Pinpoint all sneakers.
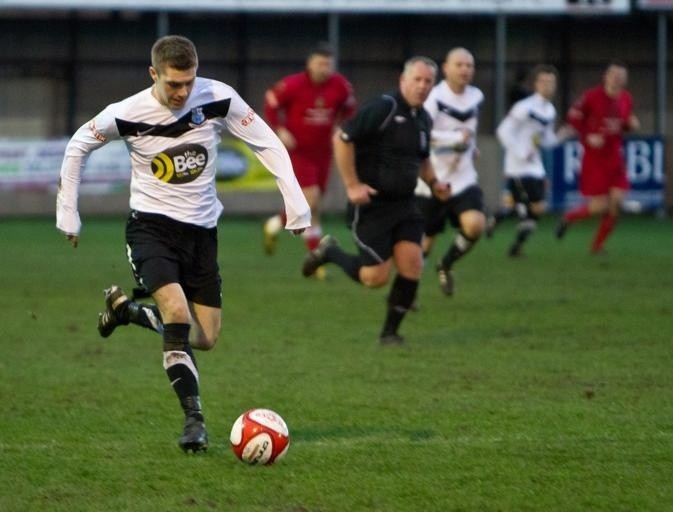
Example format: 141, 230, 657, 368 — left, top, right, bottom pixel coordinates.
263, 221, 417, 345
97, 285, 129, 338
435, 258, 454, 296
178, 412, 208, 454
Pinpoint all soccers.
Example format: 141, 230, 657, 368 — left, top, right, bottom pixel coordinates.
231, 408, 289, 466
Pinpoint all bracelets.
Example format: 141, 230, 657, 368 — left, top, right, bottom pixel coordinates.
427, 178, 437, 189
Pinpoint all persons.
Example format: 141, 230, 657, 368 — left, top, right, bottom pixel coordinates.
302, 56, 452, 349
551, 64, 643, 256
56, 36, 313, 453
409, 45, 484, 312
486, 65, 576, 260
261, 50, 359, 282
